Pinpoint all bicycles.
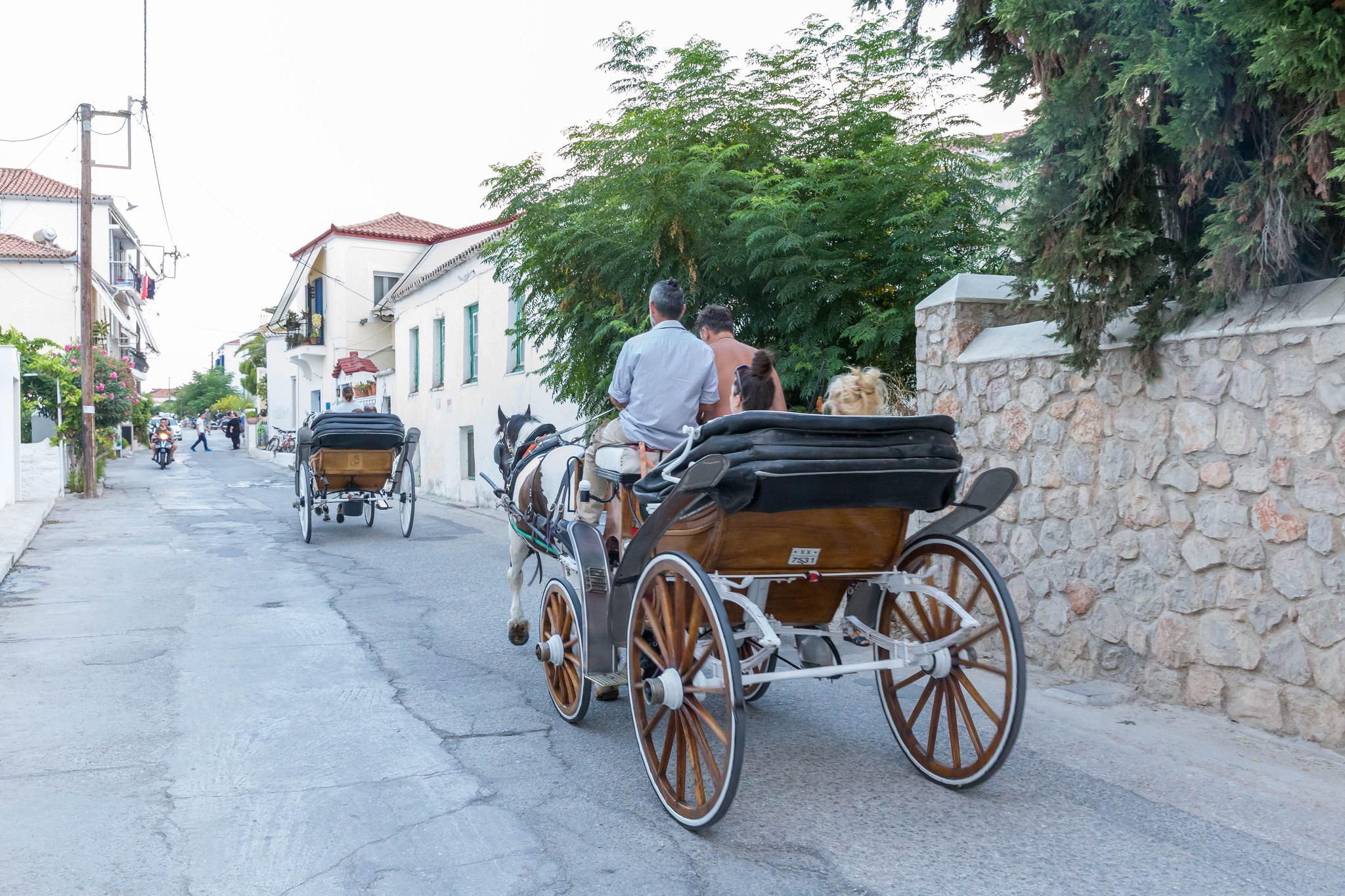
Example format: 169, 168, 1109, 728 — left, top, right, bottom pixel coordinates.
265, 426, 298, 454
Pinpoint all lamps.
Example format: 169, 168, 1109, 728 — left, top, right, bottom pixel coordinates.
336, 385, 340, 397
360, 318, 368, 325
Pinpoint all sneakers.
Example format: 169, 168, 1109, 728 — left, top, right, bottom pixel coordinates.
190, 447, 197, 452
204, 448, 212, 452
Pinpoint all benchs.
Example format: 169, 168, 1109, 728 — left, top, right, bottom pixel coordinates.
309, 448, 394, 491
639, 404, 919, 572
598, 445, 669, 495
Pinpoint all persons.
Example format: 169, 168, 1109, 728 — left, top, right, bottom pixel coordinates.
240, 411, 246, 446
227, 411, 241, 450
156, 412, 163, 417
793, 574, 871, 648
557, 277, 720, 567
821, 369, 887, 416
695, 304, 787, 427
183, 415, 190, 426
729, 350, 776, 415
150, 418, 177, 461
352, 405, 378, 413
204, 408, 212, 436
334, 386, 365, 413
190, 412, 212, 451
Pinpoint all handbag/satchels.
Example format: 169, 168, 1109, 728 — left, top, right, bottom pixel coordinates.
238, 418, 243, 433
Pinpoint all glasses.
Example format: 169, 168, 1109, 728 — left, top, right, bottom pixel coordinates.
734, 365, 752, 402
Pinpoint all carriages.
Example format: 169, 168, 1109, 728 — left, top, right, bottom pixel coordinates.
476, 402, 1027, 831
292, 408, 420, 544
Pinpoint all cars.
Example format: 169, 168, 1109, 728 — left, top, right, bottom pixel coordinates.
159, 412, 178, 423
150, 417, 182, 441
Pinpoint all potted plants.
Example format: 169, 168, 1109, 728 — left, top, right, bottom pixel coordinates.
363, 382, 373, 396
352, 382, 360, 396
284, 333, 310, 348
307, 324, 320, 344
358, 381, 368, 398
366, 377, 376, 395
300, 307, 310, 318
246, 411, 258, 424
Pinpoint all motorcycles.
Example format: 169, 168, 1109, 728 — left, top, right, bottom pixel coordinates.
191, 407, 243, 433
149, 428, 180, 470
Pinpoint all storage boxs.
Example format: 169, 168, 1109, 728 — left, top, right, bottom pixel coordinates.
116, 281, 132, 286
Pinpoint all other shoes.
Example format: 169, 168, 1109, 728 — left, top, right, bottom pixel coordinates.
209, 434, 211, 435
231, 448, 236, 450
151, 457, 155, 459
171, 459, 174, 461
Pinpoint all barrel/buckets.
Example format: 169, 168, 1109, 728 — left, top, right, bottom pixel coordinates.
341, 484, 364, 517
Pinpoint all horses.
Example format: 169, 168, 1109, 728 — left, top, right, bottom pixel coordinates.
494, 404, 620, 701
303, 409, 344, 523
